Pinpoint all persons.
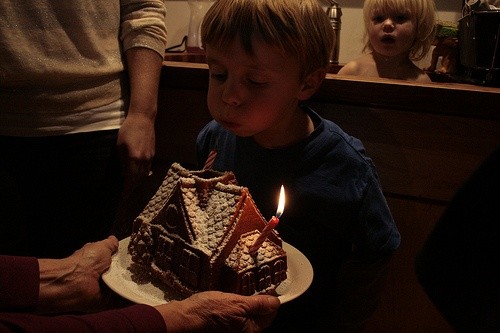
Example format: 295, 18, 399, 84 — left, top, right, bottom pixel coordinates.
0, 235, 279, 333
0, 0, 168, 261
195, 0, 401, 333
337, 0, 437, 83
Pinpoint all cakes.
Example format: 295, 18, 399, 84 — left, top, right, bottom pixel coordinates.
126, 163, 290, 303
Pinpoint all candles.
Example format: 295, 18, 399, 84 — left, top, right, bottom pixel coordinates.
247, 185, 285, 254
205, 148, 219, 170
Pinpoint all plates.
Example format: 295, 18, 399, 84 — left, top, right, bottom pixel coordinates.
101, 235, 314, 307
